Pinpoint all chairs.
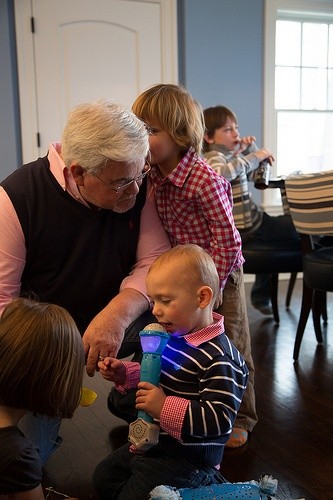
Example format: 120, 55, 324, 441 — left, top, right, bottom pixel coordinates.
228, 165, 333, 361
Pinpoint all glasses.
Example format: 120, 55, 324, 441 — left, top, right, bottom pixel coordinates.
94, 158, 151, 193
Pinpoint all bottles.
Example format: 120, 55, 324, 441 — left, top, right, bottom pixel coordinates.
252, 159, 272, 190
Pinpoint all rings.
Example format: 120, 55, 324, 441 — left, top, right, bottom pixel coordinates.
99, 356, 105, 361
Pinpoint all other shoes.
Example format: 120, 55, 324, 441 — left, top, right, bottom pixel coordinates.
107, 387, 138, 423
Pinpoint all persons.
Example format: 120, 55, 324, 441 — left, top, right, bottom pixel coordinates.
0, 299, 85, 500
92, 243, 250, 499
201, 105, 333, 315
0, 100, 171, 471
130, 83, 259, 447
